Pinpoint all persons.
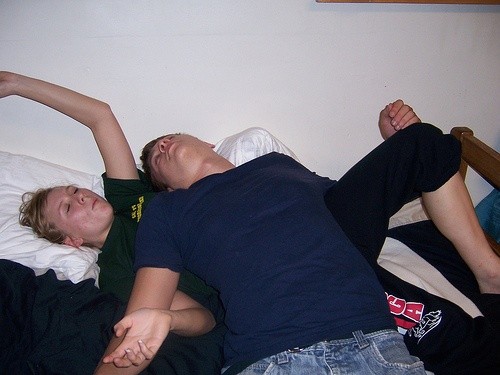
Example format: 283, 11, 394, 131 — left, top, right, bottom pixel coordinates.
102, 99, 431, 375
1, 70, 500, 375
